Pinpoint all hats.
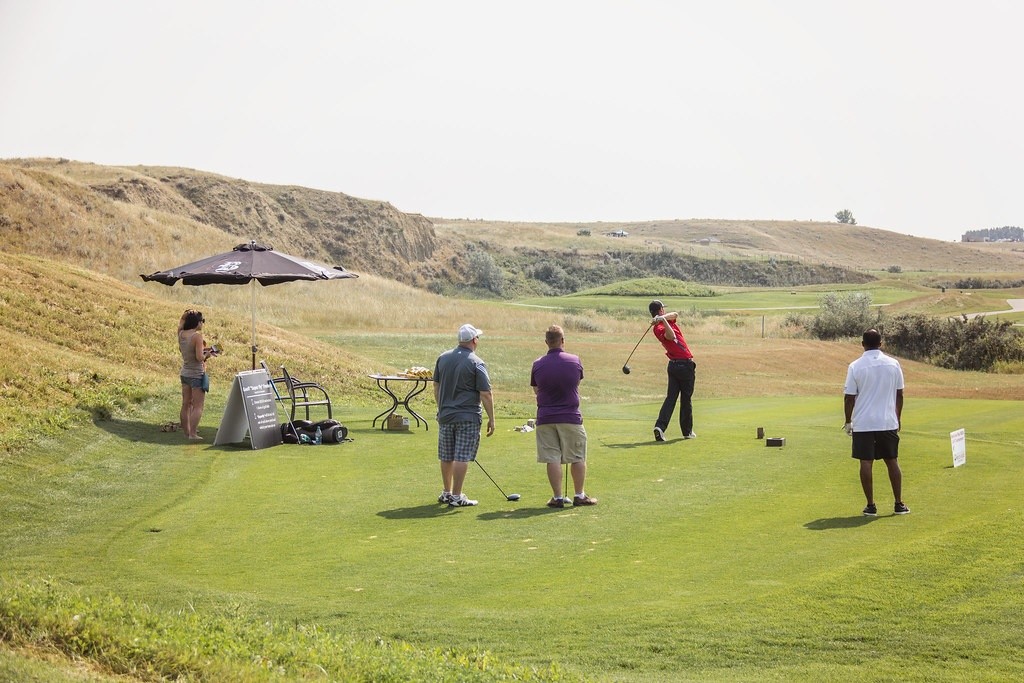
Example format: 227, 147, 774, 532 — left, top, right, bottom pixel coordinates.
649, 300, 667, 311
458, 324, 482, 342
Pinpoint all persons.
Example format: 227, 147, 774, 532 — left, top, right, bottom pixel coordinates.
844, 329, 910, 516
530, 325, 597, 507
433, 324, 495, 507
178, 309, 222, 440
649, 300, 697, 442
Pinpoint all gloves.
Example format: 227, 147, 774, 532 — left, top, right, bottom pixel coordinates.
844, 422, 852, 436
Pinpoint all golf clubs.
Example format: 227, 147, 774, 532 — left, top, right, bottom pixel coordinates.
436, 412, 522, 500
622, 314, 658, 374
259, 360, 301, 445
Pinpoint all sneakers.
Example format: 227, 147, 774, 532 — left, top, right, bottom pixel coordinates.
438, 491, 451, 503
684, 431, 696, 438
448, 495, 479, 506
894, 502, 910, 514
573, 496, 598, 506
863, 504, 877, 515
654, 427, 665, 441
546, 497, 564, 508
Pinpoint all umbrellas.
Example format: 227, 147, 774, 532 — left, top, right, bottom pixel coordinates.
139, 241, 360, 370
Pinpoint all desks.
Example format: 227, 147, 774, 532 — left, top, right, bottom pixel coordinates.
367, 375, 434, 431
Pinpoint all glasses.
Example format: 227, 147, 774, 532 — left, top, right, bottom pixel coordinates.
199, 318, 205, 323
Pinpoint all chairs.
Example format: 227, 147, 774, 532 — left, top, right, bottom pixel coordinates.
260, 361, 310, 421
279, 365, 332, 422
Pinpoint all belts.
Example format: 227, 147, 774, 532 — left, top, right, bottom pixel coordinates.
670, 358, 694, 363
